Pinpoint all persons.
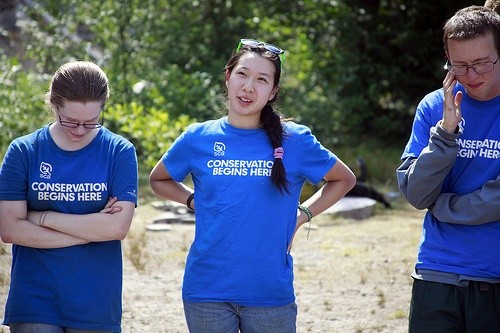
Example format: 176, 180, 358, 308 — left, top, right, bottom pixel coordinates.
396, 5, 500, 333
148, 35, 357, 333
0, 60, 138, 333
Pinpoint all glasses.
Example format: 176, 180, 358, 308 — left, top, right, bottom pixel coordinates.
59, 110, 104, 128
445, 55, 500, 75
237, 39, 284, 62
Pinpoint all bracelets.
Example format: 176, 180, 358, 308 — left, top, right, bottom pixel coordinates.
297, 200, 313, 222
38, 209, 50, 228
186, 190, 195, 210
